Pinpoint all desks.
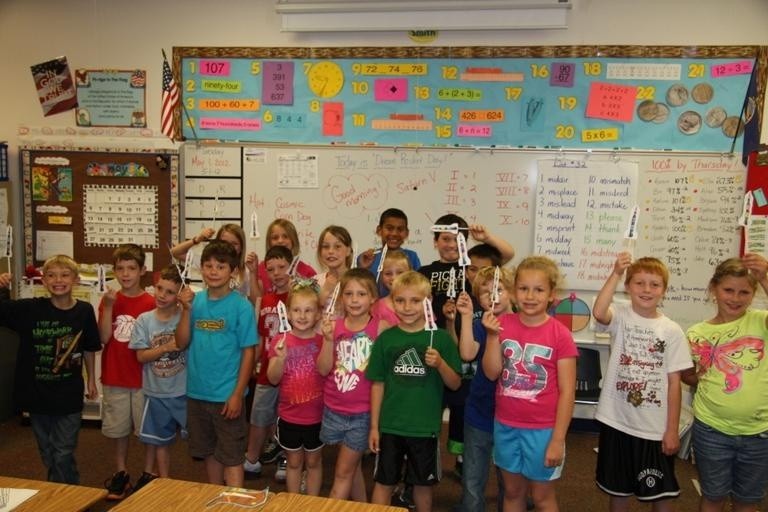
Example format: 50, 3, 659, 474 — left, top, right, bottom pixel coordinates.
0, 476, 109, 512
109, 475, 409, 512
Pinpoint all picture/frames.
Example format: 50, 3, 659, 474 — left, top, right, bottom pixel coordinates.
74, 69, 147, 127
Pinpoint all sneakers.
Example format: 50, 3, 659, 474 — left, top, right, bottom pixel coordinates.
132, 472, 159, 496
192, 448, 204, 459
107, 470, 130, 498
454, 458, 463, 479
244, 438, 308, 495
399, 482, 416, 508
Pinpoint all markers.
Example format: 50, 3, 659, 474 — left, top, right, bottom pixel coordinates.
360, 141, 378, 147
490, 143, 511, 149
331, 141, 351, 146
654, 148, 673, 151
613, 145, 632, 150
433, 143, 451, 147
453, 142, 477, 148
518, 144, 537, 149
405, 142, 423, 147
544, 144, 564, 150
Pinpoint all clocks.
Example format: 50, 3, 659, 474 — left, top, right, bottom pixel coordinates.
308, 61, 344, 97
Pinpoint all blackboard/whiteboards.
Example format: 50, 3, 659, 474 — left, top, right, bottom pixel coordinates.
178, 140, 768, 343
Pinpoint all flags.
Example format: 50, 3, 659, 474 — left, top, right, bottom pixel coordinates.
160, 58, 182, 144
742, 60, 760, 166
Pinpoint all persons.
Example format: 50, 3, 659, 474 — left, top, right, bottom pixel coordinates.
682, 253, 768, 512
592, 251, 694, 512
1, 255, 102, 485
96, 209, 579, 511
